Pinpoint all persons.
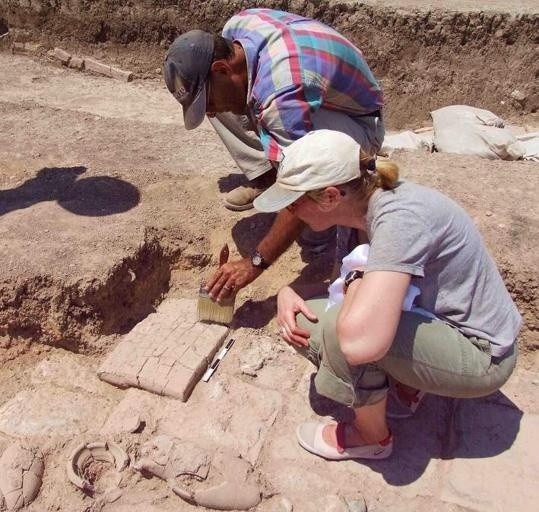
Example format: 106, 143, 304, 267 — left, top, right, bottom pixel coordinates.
271, 129, 524, 461
164, 7, 385, 304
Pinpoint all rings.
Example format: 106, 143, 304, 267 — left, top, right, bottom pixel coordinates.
279, 327, 286, 334
223, 283, 231, 292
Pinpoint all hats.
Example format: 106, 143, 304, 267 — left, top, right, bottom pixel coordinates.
253, 128, 368, 214
162, 29, 214, 129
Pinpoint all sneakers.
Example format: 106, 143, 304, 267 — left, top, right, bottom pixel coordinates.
300, 224, 339, 245
296, 418, 394, 461
224, 172, 276, 212
387, 379, 426, 420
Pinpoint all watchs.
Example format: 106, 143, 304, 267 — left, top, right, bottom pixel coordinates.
248, 248, 269, 271
343, 269, 365, 287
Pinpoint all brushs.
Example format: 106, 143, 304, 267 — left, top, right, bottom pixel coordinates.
197, 242, 237, 323
